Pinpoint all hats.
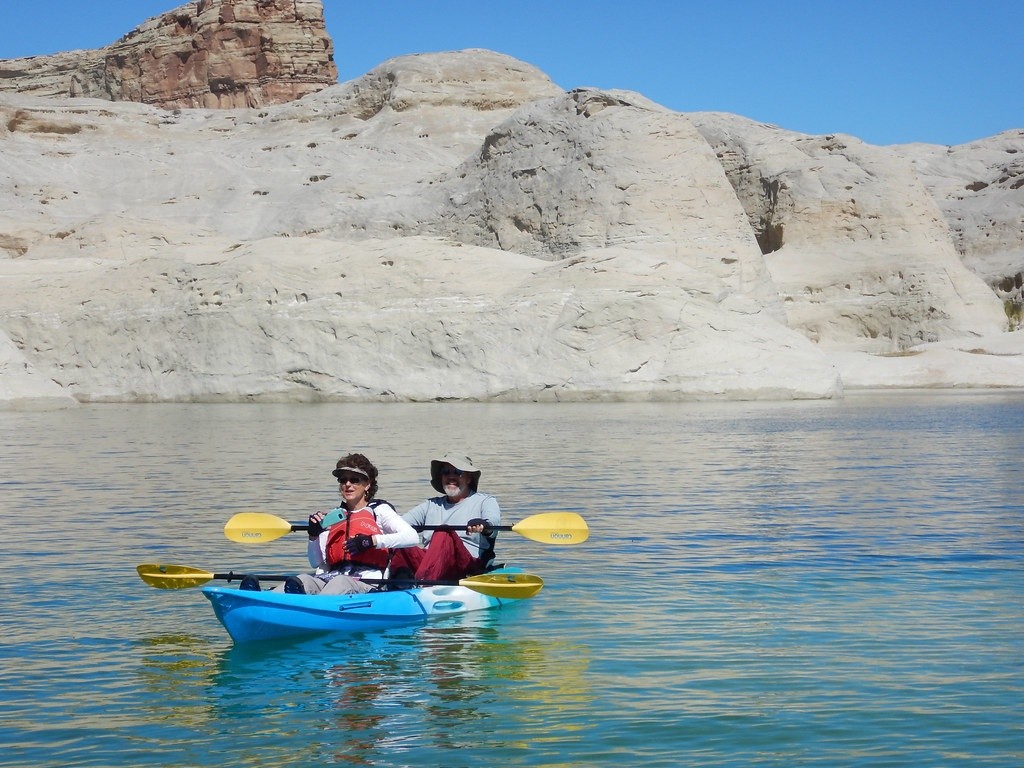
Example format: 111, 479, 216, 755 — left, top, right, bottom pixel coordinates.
430, 450, 481, 494
332, 463, 369, 483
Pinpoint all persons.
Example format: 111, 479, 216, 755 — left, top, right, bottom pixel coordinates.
389, 453, 501, 588
239, 453, 419, 594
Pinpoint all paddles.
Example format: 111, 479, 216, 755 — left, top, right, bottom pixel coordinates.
224, 510, 590, 546
136, 562, 544, 600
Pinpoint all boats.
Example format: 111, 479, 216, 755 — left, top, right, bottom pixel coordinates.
201, 568, 530, 644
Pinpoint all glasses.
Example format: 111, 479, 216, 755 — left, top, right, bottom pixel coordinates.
337, 477, 369, 484
439, 466, 470, 477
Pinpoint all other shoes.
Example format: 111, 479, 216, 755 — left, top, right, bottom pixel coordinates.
239, 575, 261, 591
389, 568, 413, 591
284, 576, 305, 594
